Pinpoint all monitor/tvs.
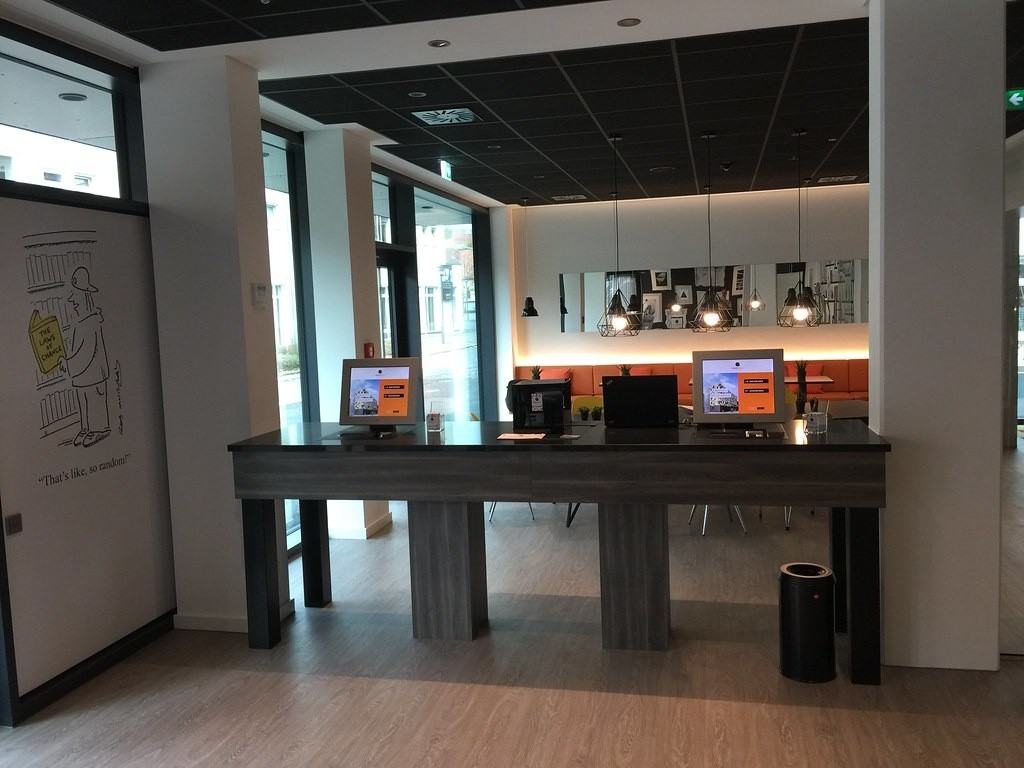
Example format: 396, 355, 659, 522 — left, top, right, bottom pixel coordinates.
692, 349, 788, 440
340, 357, 418, 440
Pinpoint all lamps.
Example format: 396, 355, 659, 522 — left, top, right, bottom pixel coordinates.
785, 281, 799, 307
744, 265, 766, 312
686, 191, 735, 333
626, 272, 641, 314
596, 196, 642, 338
519, 198, 539, 317
777, 186, 823, 328
809, 282, 831, 325
663, 270, 686, 316
798, 188, 819, 308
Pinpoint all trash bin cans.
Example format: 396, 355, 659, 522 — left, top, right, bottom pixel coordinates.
776, 563, 838, 684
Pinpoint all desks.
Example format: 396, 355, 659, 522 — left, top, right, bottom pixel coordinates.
688, 375, 834, 387
787, 400, 869, 421
226, 418, 891, 685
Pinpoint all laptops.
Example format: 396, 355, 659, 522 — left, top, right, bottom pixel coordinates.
602, 374, 679, 429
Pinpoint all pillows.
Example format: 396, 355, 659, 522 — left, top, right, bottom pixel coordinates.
538, 368, 571, 379
786, 366, 826, 394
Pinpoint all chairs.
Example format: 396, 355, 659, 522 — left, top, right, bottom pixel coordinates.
571, 397, 605, 416
679, 405, 733, 536
783, 386, 797, 405
470, 412, 535, 520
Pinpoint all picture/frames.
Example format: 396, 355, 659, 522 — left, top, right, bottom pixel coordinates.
732, 266, 744, 296
695, 267, 725, 287
651, 269, 672, 290
675, 286, 693, 305
696, 290, 730, 312
642, 293, 663, 323
737, 298, 744, 317
665, 309, 687, 329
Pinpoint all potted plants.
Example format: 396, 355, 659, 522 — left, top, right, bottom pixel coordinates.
617, 364, 634, 376
591, 406, 604, 420
794, 393, 806, 412
577, 407, 590, 420
529, 365, 543, 379
810, 396, 819, 411
794, 359, 808, 378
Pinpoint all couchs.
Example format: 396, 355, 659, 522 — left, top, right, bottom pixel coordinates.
593, 363, 674, 399
515, 365, 593, 402
675, 364, 692, 406
784, 360, 854, 413
849, 359, 870, 412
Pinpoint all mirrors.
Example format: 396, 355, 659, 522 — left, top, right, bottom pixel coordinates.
559, 260, 870, 333
